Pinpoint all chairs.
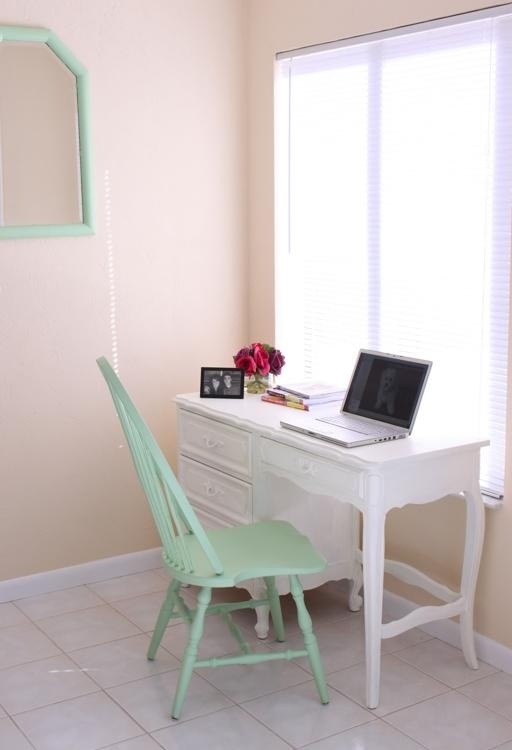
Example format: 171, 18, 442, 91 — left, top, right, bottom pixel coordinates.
96, 357, 332, 720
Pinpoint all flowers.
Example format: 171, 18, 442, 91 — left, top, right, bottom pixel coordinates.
233, 343, 284, 393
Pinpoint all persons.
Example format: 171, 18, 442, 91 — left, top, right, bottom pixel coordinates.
204, 372, 240, 396
206, 373, 225, 396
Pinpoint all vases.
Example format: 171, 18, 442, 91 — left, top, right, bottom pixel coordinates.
244, 376, 273, 391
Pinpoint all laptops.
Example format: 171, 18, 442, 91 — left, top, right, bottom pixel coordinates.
279, 347, 433, 449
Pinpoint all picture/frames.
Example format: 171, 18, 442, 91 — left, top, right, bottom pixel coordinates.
199, 367, 245, 398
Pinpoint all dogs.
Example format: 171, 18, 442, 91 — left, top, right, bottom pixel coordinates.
373, 367, 399, 416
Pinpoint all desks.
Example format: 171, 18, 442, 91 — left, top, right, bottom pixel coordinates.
172, 379, 496, 711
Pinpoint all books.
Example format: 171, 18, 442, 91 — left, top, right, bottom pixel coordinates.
261, 393, 344, 412
266, 387, 344, 404
277, 381, 348, 399
